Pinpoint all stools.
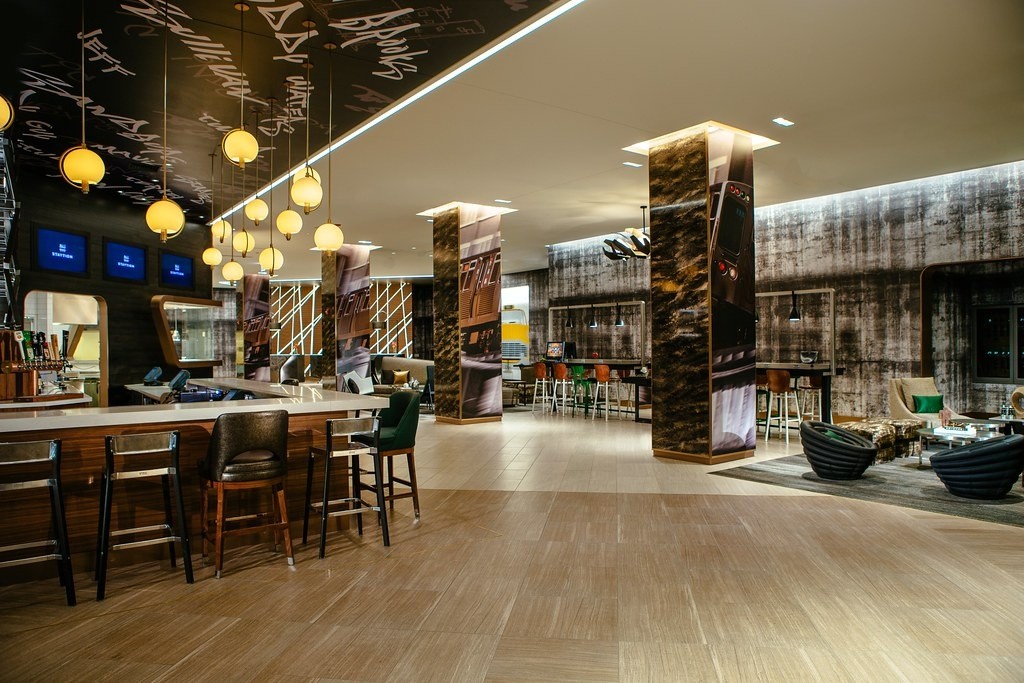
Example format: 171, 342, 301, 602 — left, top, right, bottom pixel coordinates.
504, 358, 833, 444
0, 380, 419, 606
833, 418, 928, 465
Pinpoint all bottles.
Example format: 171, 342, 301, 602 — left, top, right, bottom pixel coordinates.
1001, 405, 1007, 419
410, 377, 414, 386
1009, 405, 1013, 419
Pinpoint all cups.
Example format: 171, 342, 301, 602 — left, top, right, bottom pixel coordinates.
403, 383, 408, 388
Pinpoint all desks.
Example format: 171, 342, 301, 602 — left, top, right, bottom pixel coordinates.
124, 382, 171, 404
915, 428, 1005, 466
0, 378, 390, 588
546, 359, 648, 422
756, 363, 831, 424
988, 416, 1024, 435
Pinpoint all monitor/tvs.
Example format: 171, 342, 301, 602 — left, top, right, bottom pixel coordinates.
546, 340, 565, 361
158, 248, 196, 292
30, 222, 91, 278
102, 236, 149, 285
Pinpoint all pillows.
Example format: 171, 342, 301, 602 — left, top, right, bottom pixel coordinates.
901, 377, 939, 412
381, 369, 410, 385
912, 395, 943, 413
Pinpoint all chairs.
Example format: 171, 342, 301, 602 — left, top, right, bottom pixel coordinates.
421, 365, 435, 413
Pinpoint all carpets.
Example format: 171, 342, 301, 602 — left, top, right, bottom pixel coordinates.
708, 439, 1024, 528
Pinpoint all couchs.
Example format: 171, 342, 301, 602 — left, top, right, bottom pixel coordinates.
800, 420, 878, 480
890, 377, 1024, 499
372, 356, 435, 394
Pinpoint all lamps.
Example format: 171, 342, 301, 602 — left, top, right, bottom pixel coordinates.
172, 309, 181, 341
564, 205, 801, 328
0, 0, 344, 287
